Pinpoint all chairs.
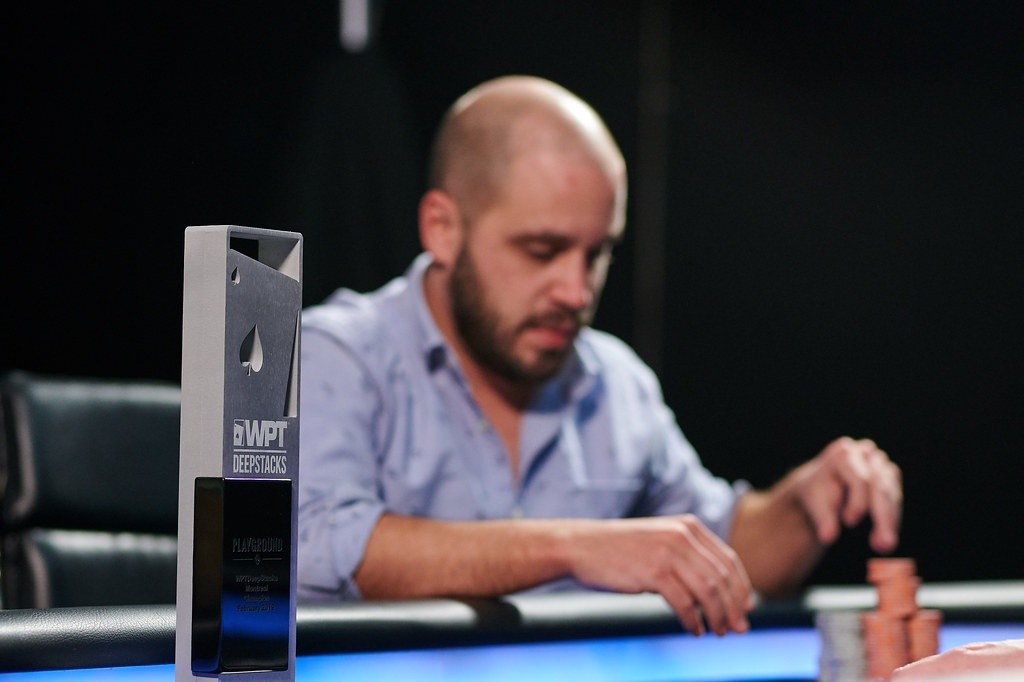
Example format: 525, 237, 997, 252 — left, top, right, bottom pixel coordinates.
0, 373, 205, 608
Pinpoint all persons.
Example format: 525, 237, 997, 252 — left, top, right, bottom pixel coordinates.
294, 72, 902, 639
